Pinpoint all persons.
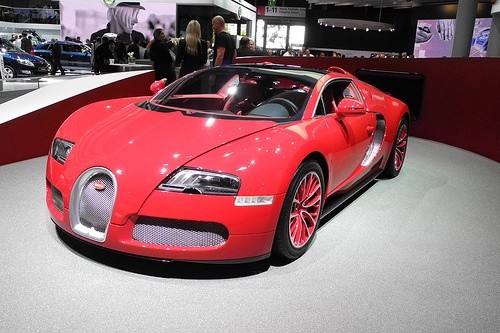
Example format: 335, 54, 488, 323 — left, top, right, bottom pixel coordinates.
175, 20, 208, 78
90, 0, 145, 41
144, 29, 176, 86
10, 25, 414, 74
416, 19, 453, 44
209, 16, 237, 94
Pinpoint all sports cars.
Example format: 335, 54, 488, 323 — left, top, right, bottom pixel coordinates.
46, 64, 411, 265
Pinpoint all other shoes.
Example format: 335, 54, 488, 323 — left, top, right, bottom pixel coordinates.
50, 73, 55, 75
61, 73, 65, 75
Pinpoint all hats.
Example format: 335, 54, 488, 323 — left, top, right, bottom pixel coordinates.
103, 0, 146, 10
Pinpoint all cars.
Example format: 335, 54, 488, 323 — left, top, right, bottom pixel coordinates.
23, 30, 94, 73
0, 38, 48, 79
